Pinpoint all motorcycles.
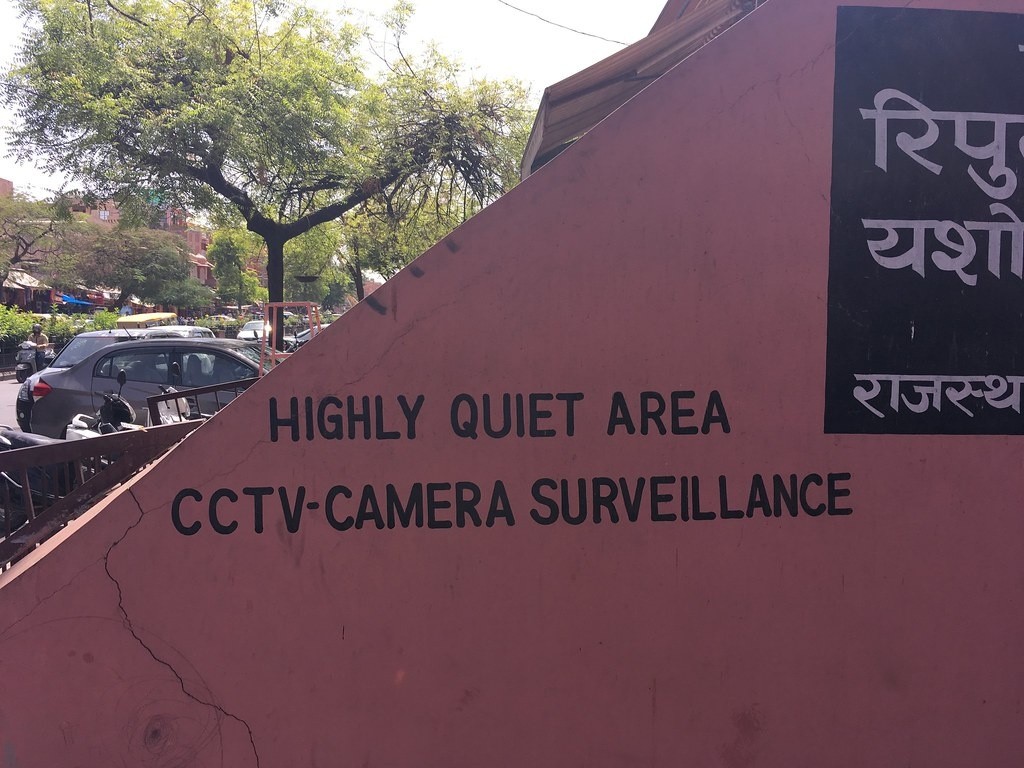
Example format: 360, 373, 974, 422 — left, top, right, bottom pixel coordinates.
116, 312, 178, 331
0, 369, 147, 521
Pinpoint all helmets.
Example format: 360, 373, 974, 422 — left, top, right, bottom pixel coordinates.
32, 323, 43, 333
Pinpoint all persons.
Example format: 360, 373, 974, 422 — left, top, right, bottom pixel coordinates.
28, 324, 49, 372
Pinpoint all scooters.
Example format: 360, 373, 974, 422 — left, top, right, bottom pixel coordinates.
142, 361, 195, 424
16, 340, 58, 383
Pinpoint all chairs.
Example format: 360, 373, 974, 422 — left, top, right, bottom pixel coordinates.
101, 361, 165, 382
185, 354, 212, 386
216, 358, 236, 384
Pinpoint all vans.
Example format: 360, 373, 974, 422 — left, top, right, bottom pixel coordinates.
147, 326, 218, 372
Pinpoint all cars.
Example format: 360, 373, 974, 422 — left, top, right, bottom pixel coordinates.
235, 320, 270, 343
30, 337, 295, 439
296, 324, 331, 342
15, 329, 192, 435
205, 316, 236, 323
